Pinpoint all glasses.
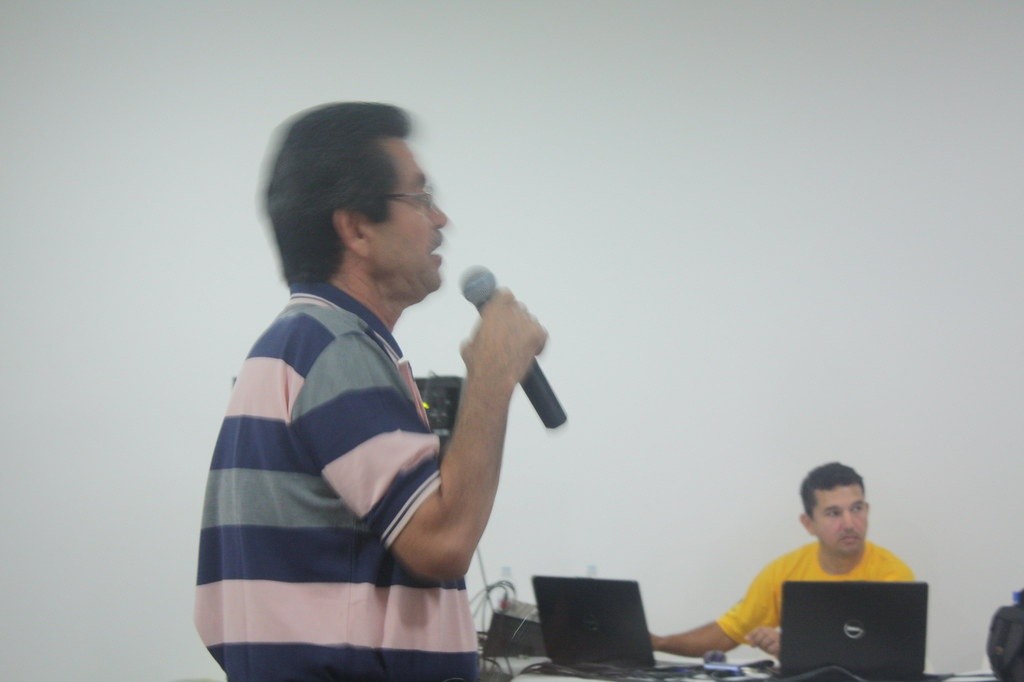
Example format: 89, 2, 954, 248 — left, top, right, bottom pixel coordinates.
339, 185, 435, 211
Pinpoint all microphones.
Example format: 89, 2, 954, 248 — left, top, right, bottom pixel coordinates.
460, 265, 568, 429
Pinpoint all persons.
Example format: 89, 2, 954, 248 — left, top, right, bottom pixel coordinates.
986, 589, 1024, 682
649, 461, 917, 659
193, 100, 546, 682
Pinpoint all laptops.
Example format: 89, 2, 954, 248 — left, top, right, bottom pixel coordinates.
532, 576, 705, 672
759, 580, 930, 682
